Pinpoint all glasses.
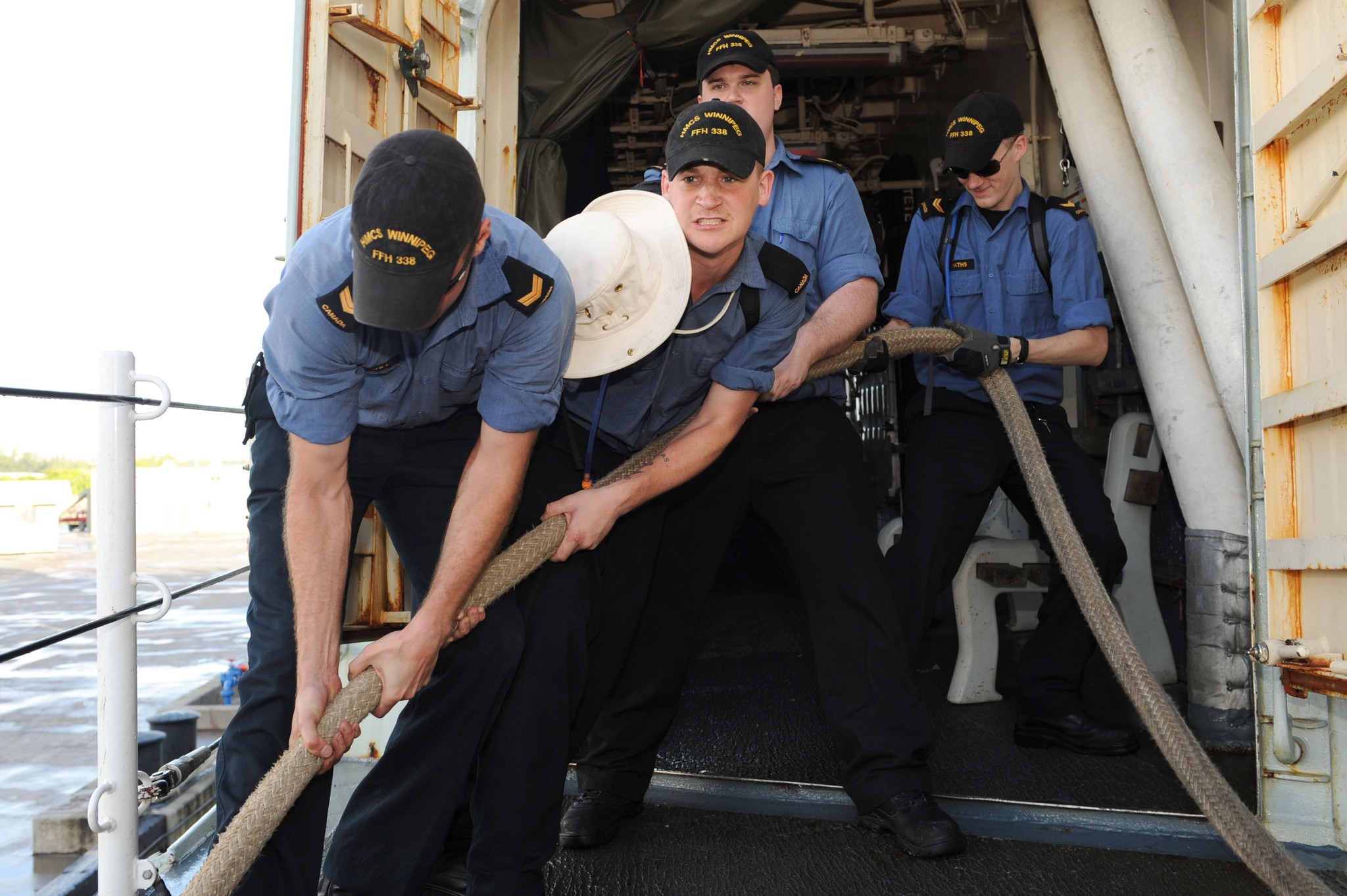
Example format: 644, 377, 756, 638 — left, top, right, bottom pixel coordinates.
949, 133, 1023, 179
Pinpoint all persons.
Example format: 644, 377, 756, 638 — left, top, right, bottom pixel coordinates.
855, 88, 1130, 859
200, 128, 580, 892
447, 100, 812, 894
548, 29, 965, 866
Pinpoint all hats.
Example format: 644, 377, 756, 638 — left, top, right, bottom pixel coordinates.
697, 29, 781, 85
350, 129, 486, 333
665, 98, 767, 182
540, 190, 693, 379
942, 89, 1024, 172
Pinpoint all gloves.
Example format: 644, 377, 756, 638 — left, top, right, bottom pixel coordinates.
847, 331, 890, 377
933, 318, 1013, 381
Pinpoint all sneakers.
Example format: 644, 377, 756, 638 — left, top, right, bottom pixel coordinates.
1013, 708, 1140, 757
558, 789, 644, 851
231, 862, 469, 896
856, 787, 962, 857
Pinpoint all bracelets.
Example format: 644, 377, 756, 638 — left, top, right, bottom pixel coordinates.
1011, 335, 1029, 367
